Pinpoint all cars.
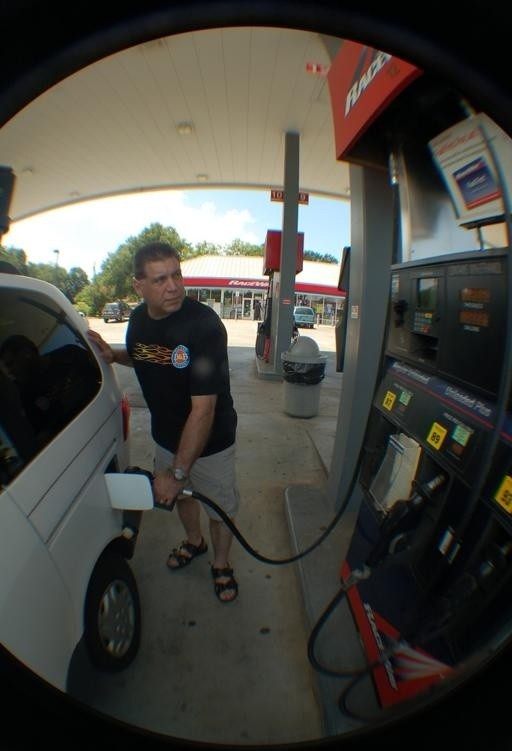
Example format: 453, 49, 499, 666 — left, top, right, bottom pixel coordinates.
1, 272, 155, 706
293, 306, 315, 328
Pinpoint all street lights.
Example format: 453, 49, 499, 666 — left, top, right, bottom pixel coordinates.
53, 249, 59, 265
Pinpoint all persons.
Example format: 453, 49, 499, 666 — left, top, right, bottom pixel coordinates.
87, 242, 241, 604
253, 300, 264, 322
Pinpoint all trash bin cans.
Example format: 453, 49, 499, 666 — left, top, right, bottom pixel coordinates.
281, 336, 328, 417
230, 310, 235, 319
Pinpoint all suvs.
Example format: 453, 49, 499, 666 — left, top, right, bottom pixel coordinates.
102, 301, 132, 323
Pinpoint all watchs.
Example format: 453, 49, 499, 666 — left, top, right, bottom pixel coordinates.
166, 465, 187, 483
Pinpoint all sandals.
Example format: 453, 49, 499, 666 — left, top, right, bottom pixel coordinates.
166, 535, 208, 569
212, 562, 237, 602
